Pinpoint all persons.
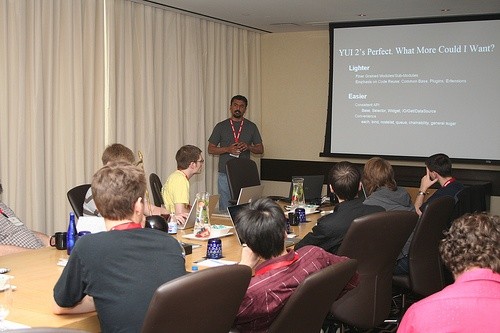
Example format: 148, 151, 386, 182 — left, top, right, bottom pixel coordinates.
294, 160, 386, 253
0, 183, 55, 256
207, 96, 264, 212
414, 153, 461, 215
160, 144, 205, 216
397, 211, 499, 333
362, 157, 419, 275
231, 196, 361, 333
52, 162, 187, 333
82, 143, 188, 226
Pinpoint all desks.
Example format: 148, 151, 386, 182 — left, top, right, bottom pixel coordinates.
0, 200, 341, 333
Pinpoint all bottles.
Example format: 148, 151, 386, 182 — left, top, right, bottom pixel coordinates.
207, 238, 223, 258
295, 208, 306, 223
66, 211, 77, 254
286, 218, 290, 233
167, 214, 178, 234
289, 212, 300, 226
191, 266, 198, 272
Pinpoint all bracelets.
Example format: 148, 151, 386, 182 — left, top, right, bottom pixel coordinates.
418, 191, 426, 196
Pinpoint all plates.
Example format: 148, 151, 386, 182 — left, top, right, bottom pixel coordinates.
284, 210, 319, 216
182, 232, 234, 241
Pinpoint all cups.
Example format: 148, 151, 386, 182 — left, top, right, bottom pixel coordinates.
291, 178, 305, 210
194, 192, 210, 237
330, 193, 338, 203
49, 232, 66, 250
78, 231, 91, 238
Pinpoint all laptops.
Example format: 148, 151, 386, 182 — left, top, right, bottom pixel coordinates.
282, 174, 325, 203
227, 202, 302, 249
177, 198, 197, 229
213, 185, 263, 218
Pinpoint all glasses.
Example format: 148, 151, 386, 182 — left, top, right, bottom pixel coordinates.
189, 159, 204, 164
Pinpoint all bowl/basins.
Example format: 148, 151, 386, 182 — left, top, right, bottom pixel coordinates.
210, 225, 234, 233
305, 205, 320, 210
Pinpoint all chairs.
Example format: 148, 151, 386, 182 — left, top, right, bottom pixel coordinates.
67, 174, 489, 333
